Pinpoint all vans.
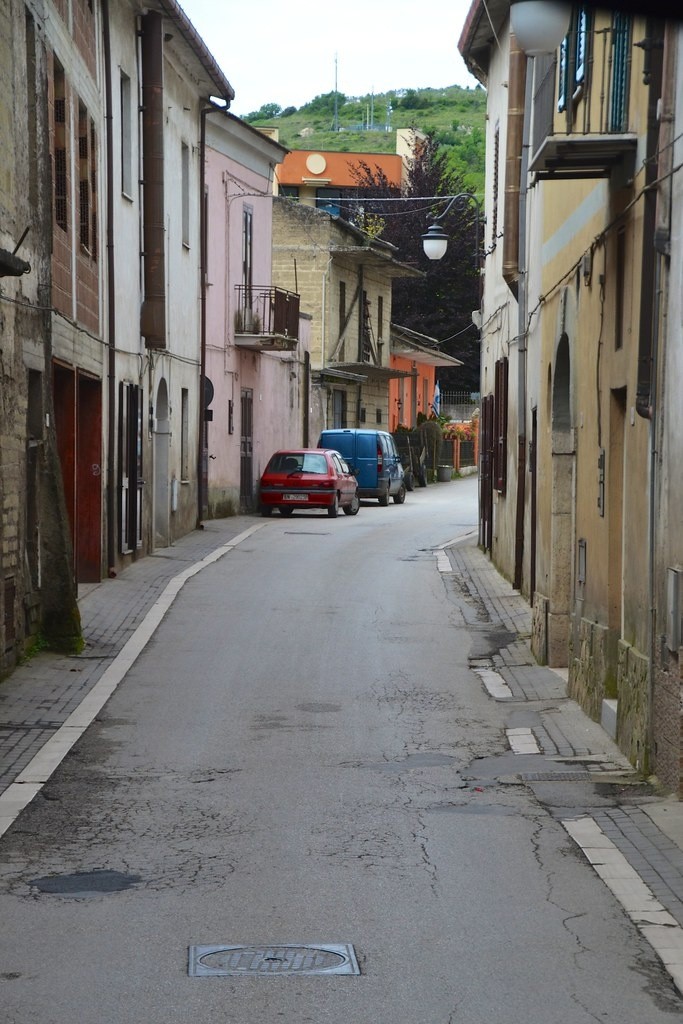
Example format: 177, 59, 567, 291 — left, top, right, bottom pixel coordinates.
317, 428, 406, 505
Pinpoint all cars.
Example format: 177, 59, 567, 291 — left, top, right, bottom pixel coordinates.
260, 447, 360, 517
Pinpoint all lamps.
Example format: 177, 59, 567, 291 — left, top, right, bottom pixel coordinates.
395, 398, 403, 411
508, 0, 571, 56
421, 192, 482, 273
